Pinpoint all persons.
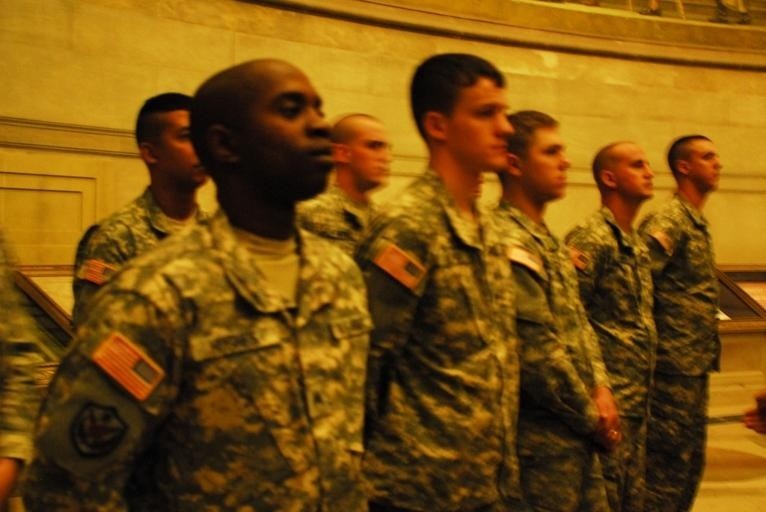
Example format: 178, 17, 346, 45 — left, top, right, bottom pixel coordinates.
1, 52, 724, 512
